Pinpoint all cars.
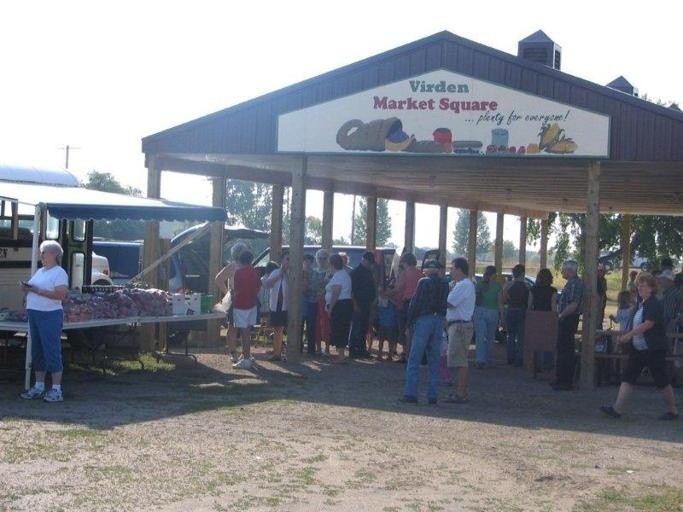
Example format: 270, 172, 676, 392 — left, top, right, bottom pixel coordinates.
441, 271, 561, 349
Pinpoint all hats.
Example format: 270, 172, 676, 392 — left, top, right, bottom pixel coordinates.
423, 258, 440, 269
363, 251, 375, 263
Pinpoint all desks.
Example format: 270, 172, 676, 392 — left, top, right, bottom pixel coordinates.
136, 312, 227, 380
0, 311, 136, 380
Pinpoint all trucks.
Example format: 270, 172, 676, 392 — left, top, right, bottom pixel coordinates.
597, 248, 655, 272
0, 166, 114, 350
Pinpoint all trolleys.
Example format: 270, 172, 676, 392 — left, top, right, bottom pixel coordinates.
570, 315, 616, 386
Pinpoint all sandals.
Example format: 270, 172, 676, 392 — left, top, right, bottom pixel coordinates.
401, 393, 472, 406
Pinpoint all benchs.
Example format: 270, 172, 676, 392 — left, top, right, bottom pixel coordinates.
568, 331, 683, 388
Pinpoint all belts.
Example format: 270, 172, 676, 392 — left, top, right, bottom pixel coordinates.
447, 320, 470, 325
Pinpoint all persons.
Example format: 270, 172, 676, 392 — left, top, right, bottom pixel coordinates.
18, 240, 69, 403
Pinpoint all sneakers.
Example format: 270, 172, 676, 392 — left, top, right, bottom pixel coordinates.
599, 406, 622, 418
657, 411, 679, 421
230, 353, 252, 369
548, 382, 569, 391
43, 389, 64, 402
20, 386, 45, 400
265, 343, 408, 367
473, 359, 554, 376
